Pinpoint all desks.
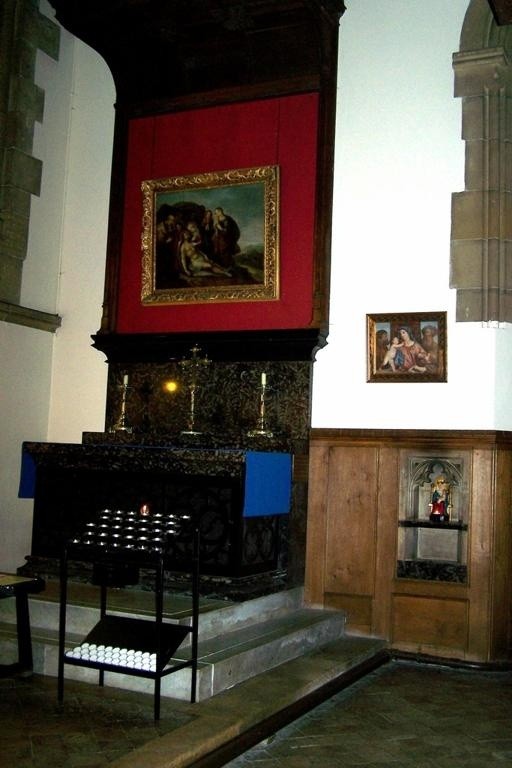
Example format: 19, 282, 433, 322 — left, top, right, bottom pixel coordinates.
0, 571, 47, 676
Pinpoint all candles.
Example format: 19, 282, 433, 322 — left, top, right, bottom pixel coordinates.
261, 373, 267, 385
124, 374, 130, 385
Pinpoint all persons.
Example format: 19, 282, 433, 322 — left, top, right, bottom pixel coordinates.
155, 207, 241, 289
428, 476, 450, 522
375, 326, 438, 372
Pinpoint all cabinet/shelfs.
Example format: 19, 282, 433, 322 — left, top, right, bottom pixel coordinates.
17, 432, 301, 598
394, 446, 470, 586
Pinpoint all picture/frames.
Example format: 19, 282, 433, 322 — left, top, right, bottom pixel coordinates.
365, 309, 448, 385
140, 164, 281, 305
